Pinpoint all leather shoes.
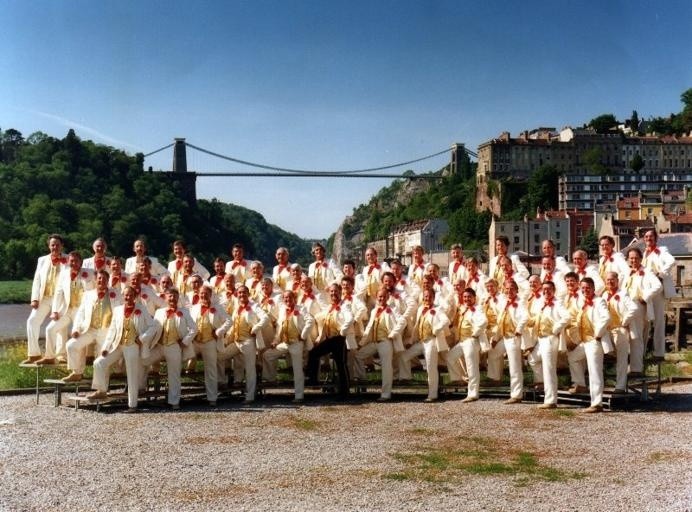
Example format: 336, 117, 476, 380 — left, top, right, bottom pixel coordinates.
120, 398, 304, 413
579, 406, 602, 413
376, 379, 438, 401
62, 373, 81, 381
21, 356, 42, 364
537, 404, 557, 408
568, 387, 588, 393
35, 359, 55, 364
87, 391, 107, 399
446, 380, 544, 404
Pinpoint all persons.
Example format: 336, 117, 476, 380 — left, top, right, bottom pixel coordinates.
23, 227, 674, 411
